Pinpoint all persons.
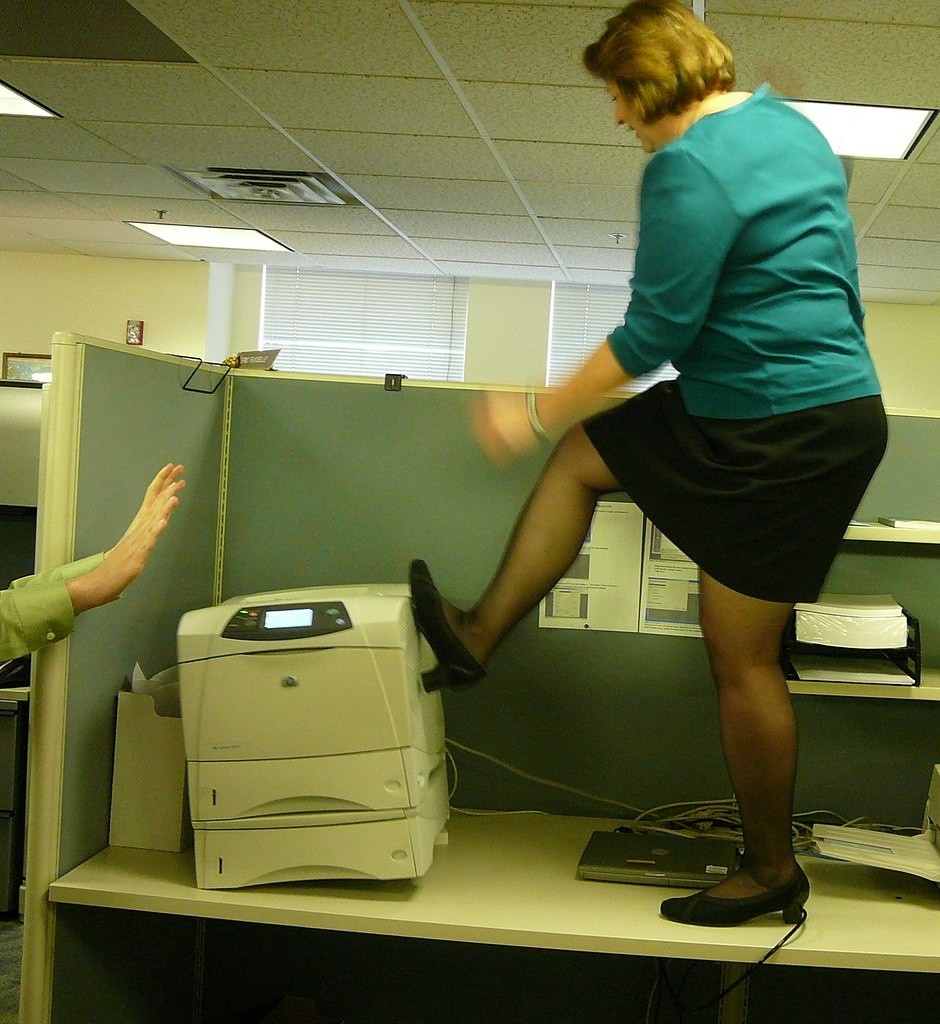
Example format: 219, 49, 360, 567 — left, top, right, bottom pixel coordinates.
1, 462, 185, 665
409, 1, 888, 928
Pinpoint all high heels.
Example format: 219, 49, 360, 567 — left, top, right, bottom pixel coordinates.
410, 559, 487, 692
660, 861, 809, 928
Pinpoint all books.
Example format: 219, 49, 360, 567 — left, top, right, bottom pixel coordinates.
877, 517, 940, 529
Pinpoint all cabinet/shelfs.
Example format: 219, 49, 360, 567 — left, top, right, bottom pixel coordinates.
784, 522, 940, 702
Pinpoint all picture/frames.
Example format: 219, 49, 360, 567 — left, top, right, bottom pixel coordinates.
2, 352, 52, 381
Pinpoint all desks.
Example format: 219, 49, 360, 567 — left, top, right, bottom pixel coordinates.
49, 813, 940, 1024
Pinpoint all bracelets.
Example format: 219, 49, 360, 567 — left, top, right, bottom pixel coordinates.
525, 390, 548, 437
